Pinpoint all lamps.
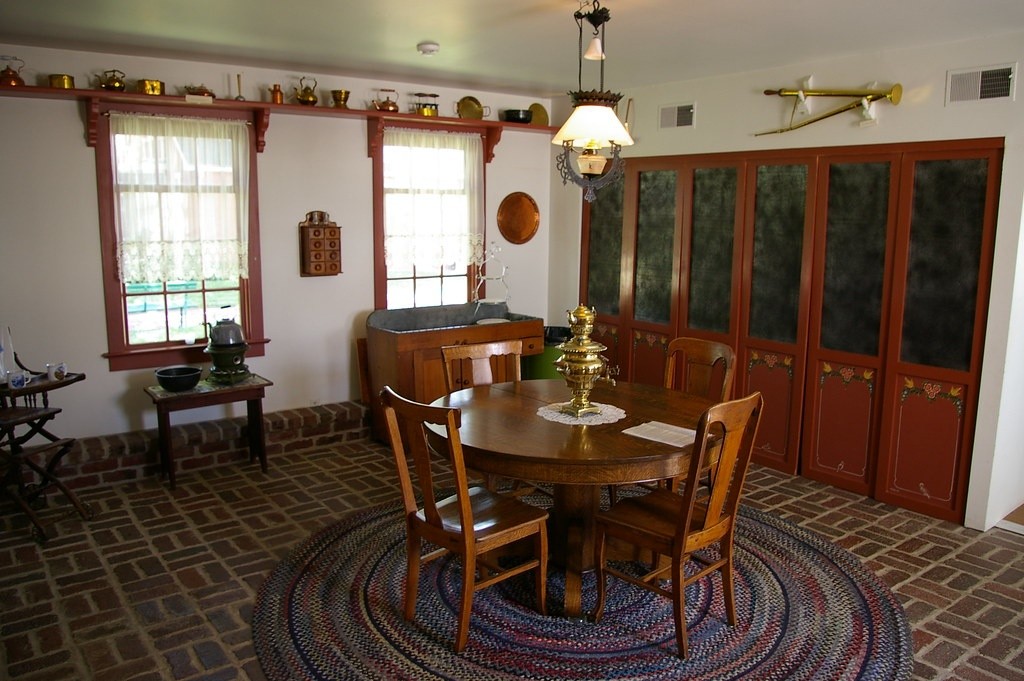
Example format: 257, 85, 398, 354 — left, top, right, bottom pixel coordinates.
549, 0, 636, 204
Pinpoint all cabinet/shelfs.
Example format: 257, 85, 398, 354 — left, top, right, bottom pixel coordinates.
365, 301, 546, 454
580, 136, 1007, 525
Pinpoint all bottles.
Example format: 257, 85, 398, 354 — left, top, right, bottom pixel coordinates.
271, 84, 284, 105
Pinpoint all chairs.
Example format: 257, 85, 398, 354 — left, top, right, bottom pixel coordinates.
380, 338, 764, 658
0, 326, 95, 539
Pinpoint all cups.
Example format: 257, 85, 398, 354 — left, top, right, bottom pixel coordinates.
46, 361, 67, 382
7, 369, 31, 389
331, 90, 350, 108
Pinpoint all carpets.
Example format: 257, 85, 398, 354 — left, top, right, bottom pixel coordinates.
247, 487, 913, 681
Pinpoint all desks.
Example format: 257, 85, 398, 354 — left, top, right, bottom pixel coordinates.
426, 379, 732, 617
144, 374, 272, 491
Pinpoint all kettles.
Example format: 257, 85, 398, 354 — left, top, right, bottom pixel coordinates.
94, 69, 126, 92
0, 54, 26, 88
207, 305, 243, 346
293, 76, 318, 106
372, 89, 399, 113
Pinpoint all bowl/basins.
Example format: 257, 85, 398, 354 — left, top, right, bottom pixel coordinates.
503, 109, 533, 123
48, 73, 75, 89
155, 365, 203, 393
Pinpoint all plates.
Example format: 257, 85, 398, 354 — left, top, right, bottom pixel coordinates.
476, 318, 511, 324
529, 103, 549, 126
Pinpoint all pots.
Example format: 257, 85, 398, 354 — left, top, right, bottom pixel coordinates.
452, 96, 491, 120
